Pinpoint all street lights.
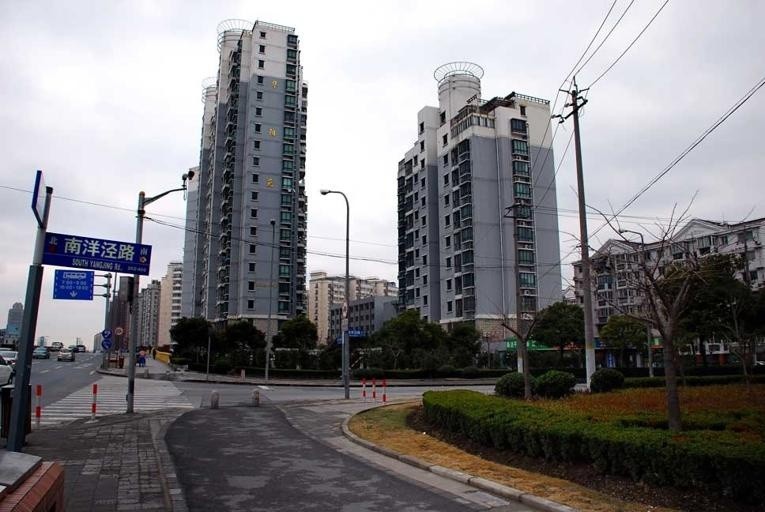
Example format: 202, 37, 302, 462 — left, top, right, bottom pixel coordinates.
320, 189, 350, 398
265, 219, 276, 381
619, 229, 654, 377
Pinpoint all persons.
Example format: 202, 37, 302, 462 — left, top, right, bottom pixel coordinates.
152, 348, 157, 360
137, 350, 146, 367
148, 344, 152, 355
5, 360, 17, 384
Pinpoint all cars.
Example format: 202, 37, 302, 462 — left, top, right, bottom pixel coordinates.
0, 348, 19, 386
32, 341, 86, 362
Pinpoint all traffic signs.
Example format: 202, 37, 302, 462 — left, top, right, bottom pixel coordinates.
41, 231, 152, 276
53, 269, 94, 300
348, 330, 370, 337
337, 337, 342, 344
